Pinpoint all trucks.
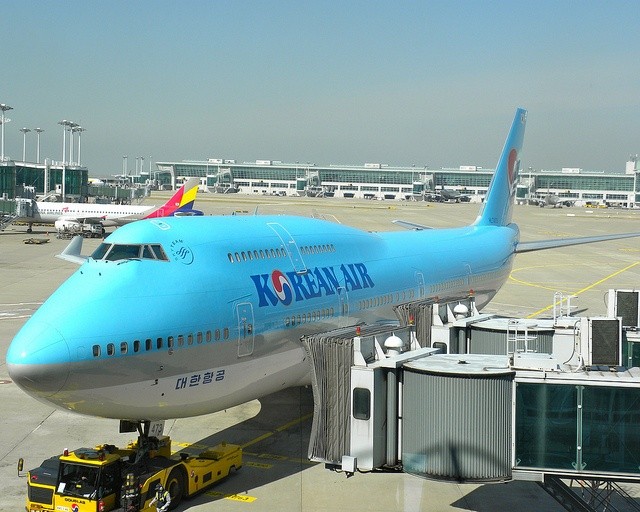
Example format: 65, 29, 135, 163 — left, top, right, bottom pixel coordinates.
54, 216, 104, 238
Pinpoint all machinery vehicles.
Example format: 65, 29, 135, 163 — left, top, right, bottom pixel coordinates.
17, 436, 242, 512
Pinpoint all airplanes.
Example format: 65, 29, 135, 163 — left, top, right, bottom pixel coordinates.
6, 106, 640, 448
0, 177, 204, 233
